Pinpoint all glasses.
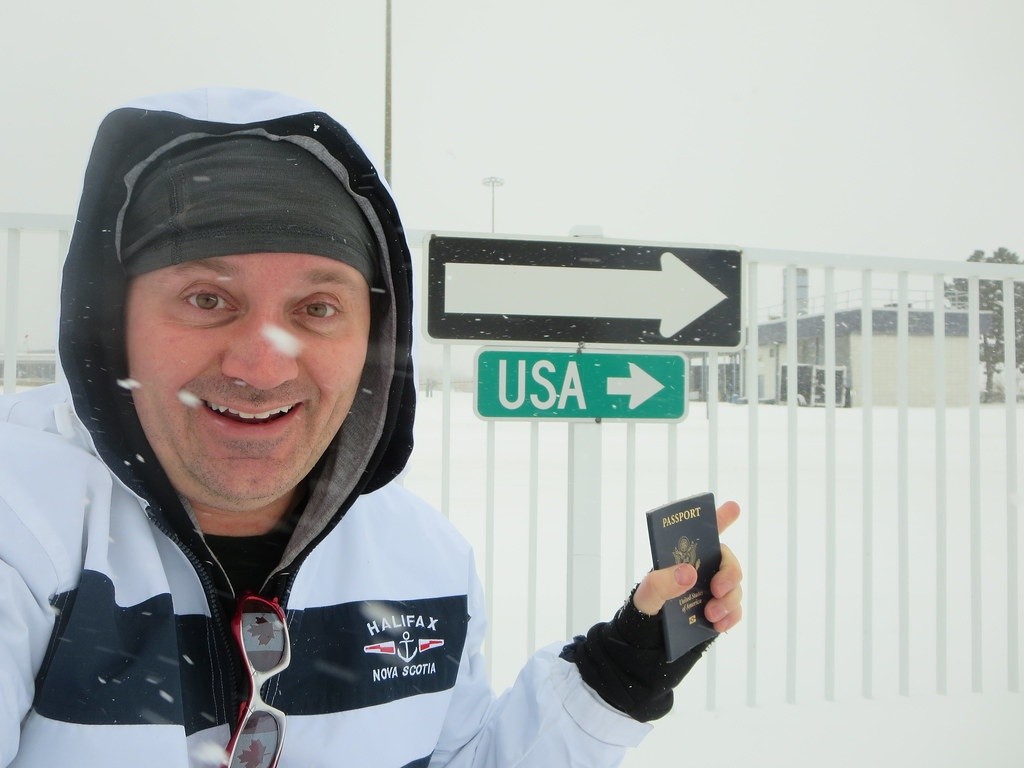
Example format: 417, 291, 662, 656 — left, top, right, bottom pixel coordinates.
219, 592, 292, 768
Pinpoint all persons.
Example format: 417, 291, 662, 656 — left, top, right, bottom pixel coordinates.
0, 87, 744, 768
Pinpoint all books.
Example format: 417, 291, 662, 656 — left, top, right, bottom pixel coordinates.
644, 492, 722, 661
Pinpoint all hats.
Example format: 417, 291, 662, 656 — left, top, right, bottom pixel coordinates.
120, 143, 373, 280
